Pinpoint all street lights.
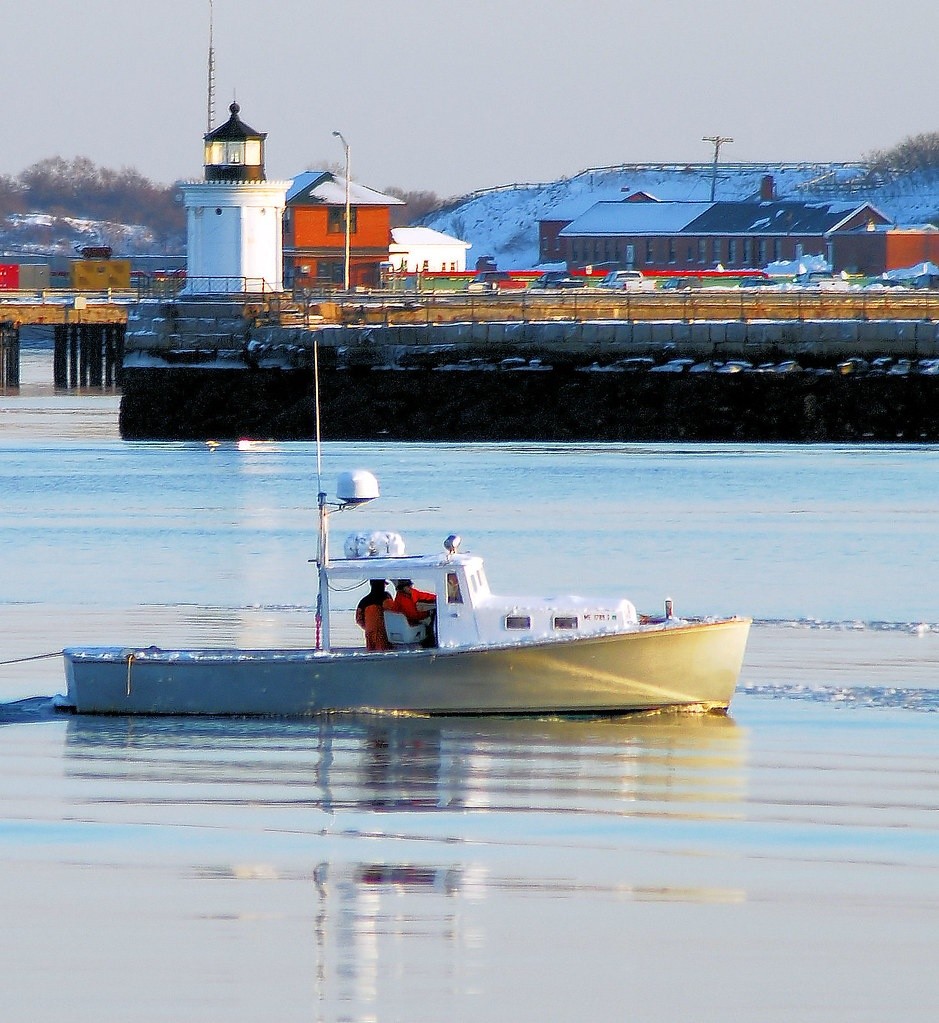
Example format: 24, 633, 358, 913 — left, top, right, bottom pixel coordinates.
332, 130, 352, 295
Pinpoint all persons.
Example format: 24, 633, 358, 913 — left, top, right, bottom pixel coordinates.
356, 579, 437, 653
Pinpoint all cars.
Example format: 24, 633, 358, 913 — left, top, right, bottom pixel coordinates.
659, 275, 703, 289
739, 276, 780, 288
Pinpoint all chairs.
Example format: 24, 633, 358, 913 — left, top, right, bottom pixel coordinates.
365, 605, 388, 651
384, 610, 426, 651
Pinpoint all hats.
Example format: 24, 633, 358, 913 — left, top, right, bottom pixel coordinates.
370, 579, 389, 586
395, 579, 413, 590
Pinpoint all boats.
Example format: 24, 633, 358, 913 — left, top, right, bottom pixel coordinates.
45, 339, 756, 716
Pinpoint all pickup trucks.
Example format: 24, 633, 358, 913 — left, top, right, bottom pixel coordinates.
790, 271, 841, 287
530, 270, 584, 288
465, 270, 527, 293
595, 270, 658, 293
